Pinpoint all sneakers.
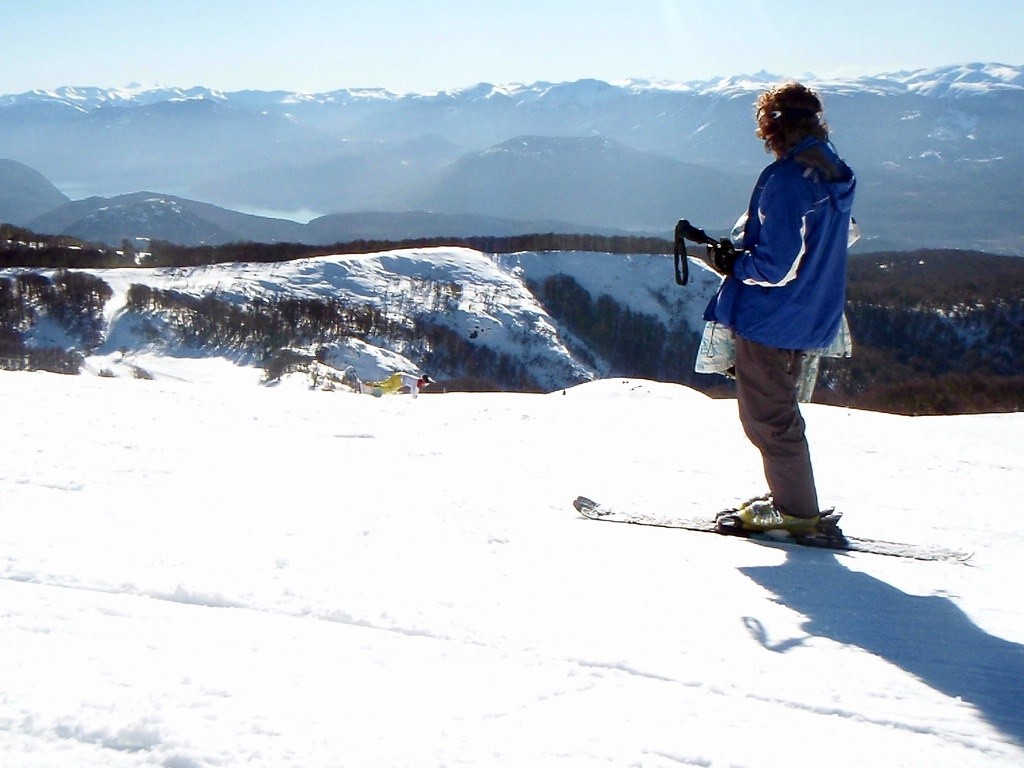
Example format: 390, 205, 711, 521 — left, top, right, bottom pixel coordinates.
734, 493, 821, 536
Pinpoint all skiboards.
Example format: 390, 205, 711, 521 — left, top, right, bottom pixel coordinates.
573, 495, 977, 563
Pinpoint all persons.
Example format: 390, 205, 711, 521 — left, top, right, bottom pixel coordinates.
703, 79, 856, 549
366, 371, 436, 399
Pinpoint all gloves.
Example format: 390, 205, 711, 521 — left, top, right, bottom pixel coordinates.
706, 236, 736, 275
